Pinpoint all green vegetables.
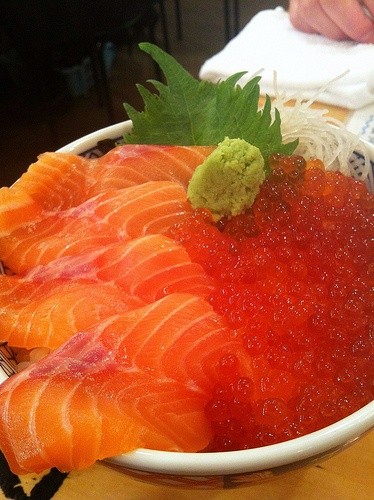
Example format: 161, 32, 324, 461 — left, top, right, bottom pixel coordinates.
122, 43, 300, 176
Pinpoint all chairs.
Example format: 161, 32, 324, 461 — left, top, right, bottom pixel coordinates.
1, 0, 238, 151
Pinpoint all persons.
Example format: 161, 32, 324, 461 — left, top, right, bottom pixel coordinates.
288, 0, 374, 49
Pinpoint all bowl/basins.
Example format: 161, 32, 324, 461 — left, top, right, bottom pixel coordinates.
47, 108, 373, 486
